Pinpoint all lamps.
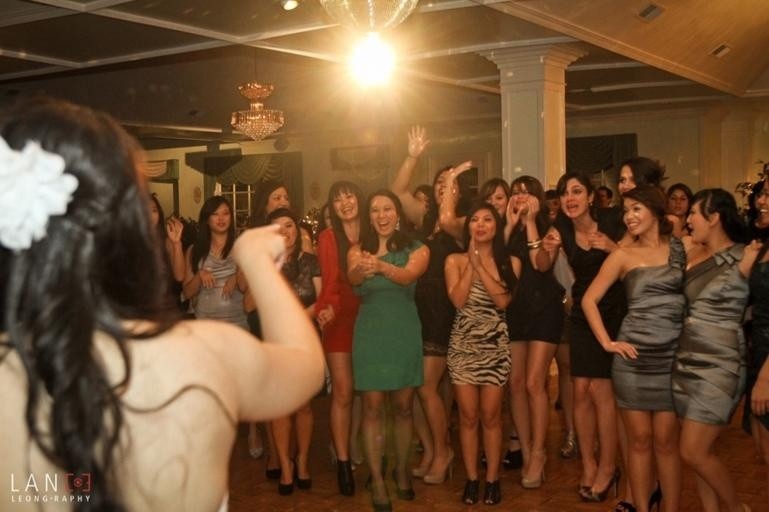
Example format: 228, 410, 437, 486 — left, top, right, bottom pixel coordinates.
229, 43, 287, 142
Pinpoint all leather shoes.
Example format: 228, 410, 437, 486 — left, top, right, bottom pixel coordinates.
247, 427, 311, 496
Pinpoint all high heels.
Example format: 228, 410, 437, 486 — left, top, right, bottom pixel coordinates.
560, 430, 662, 512
328, 421, 548, 512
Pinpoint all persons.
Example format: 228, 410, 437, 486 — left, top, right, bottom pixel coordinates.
146, 124, 769, 511
1, 94, 326, 512
